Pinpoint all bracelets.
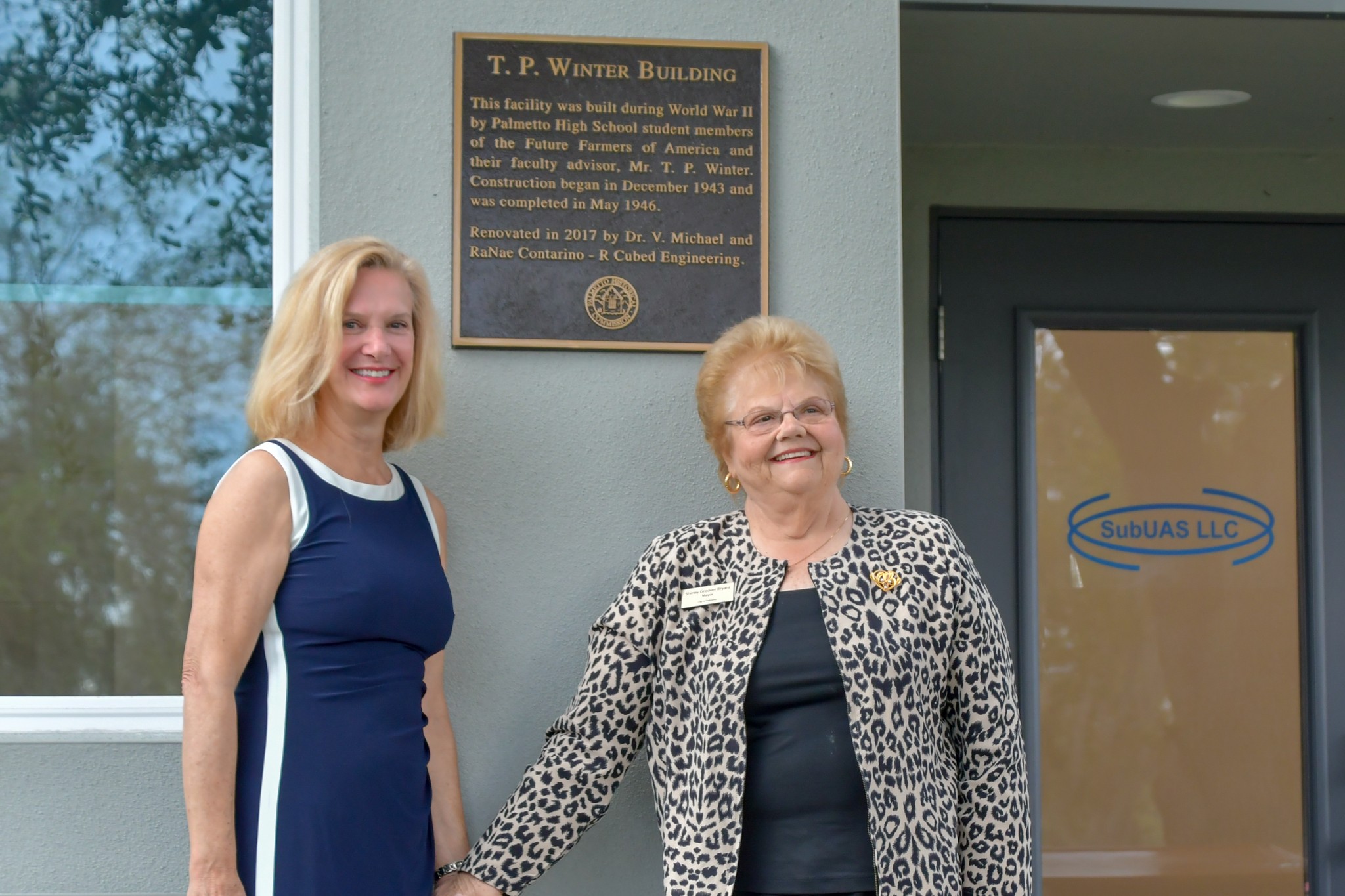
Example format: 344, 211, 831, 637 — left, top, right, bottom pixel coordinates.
434, 859, 464, 882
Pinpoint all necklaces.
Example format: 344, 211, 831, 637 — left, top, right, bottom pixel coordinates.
787, 508, 852, 569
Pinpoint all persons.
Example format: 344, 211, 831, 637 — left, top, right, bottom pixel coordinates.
418, 317, 1037, 894
179, 240, 468, 896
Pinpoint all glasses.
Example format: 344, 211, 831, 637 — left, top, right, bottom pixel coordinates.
722, 397, 838, 435
